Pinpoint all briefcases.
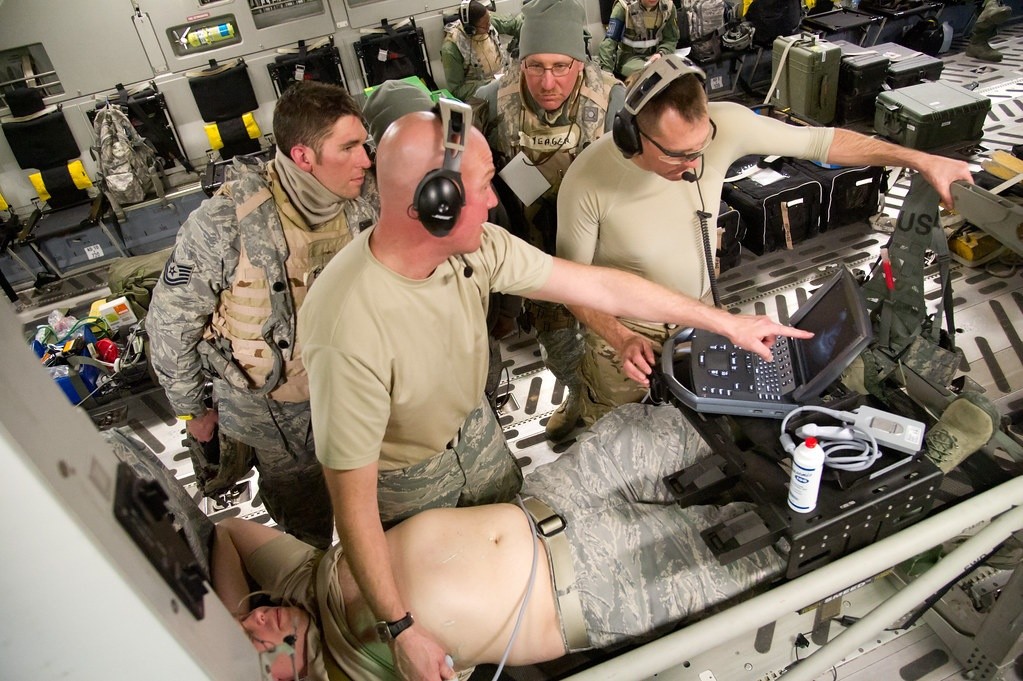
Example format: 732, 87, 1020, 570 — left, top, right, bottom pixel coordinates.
770, 30, 844, 125
833, 38, 891, 101
873, 78, 992, 151
867, 41, 944, 90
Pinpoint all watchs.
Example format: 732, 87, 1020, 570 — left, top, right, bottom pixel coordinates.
375, 611, 414, 644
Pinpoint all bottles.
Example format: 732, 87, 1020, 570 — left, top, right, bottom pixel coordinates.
787, 436, 825, 514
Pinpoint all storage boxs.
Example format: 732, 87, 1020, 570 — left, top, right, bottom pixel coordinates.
56, 324, 101, 405
98, 295, 138, 331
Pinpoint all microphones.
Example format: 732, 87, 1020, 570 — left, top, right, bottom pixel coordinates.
468, 30, 492, 41
460, 255, 473, 277
682, 155, 704, 184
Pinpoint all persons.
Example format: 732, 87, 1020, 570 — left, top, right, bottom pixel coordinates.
208, 347, 999, 681
469, 0, 631, 442
554, 51, 976, 429
147, 86, 383, 551
441, 0, 527, 106
965, 0, 1012, 62
601, 0, 680, 80
295, 107, 819, 681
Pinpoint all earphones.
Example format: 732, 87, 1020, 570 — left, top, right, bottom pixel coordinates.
413, 98, 473, 237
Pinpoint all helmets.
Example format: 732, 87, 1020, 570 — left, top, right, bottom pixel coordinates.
722, 23, 757, 52
687, 31, 722, 64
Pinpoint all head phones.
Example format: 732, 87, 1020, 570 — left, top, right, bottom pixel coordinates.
461, 0, 477, 36
614, 56, 708, 159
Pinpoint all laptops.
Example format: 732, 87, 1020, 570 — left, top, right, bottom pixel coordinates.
662, 264, 873, 418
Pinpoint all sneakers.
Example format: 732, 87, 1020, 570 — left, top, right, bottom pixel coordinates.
837, 347, 891, 400
545, 393, 582, 442
965, 41, 1003, 62
975, 0, 1013, 30
920, 389, 1000, 475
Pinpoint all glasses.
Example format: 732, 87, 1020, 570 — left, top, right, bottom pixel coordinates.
524, 58, 579, 77
474, 21, 490, 30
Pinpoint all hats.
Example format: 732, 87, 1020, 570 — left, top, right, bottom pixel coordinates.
517, 0, 587, 63
359, 78, 440, 151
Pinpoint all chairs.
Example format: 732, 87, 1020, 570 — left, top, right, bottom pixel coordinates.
685, 0, 947, 100
0, 0, 498, 302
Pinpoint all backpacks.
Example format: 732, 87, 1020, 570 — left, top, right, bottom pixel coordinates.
682, 0, 744, 47
88, 103, 166, 223
743, 0, 808, 48
